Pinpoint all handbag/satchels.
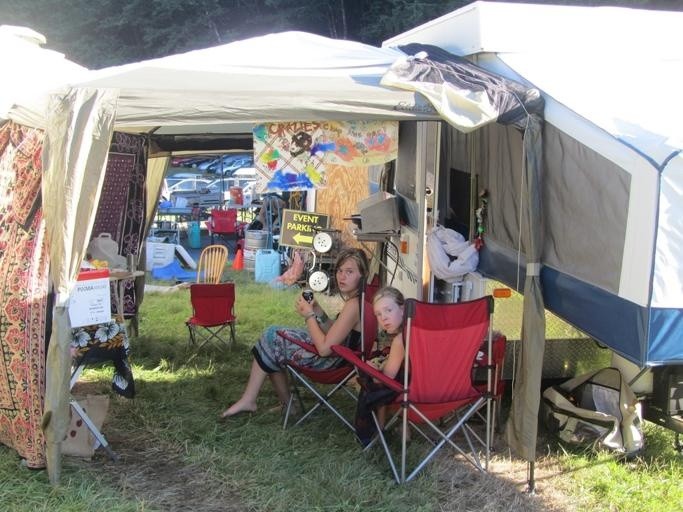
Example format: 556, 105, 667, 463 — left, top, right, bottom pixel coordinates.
541, 367, 651, 455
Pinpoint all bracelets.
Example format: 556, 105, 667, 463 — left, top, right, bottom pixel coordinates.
303, 311, 317, 323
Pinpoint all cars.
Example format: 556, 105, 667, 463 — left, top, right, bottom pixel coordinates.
163, 156, 265, 210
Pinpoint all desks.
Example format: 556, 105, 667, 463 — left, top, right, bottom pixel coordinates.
228, 200, 254, 223
69, 315, 136, 464
110, 272, 154, 339
157, 208, 193, 222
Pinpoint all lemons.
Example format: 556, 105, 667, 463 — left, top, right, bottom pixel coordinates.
93, 259, 107, 268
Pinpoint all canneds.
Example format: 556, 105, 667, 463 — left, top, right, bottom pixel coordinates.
303, 290, 314, 304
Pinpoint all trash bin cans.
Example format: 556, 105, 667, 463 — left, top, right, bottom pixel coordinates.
145, 228, 179, 272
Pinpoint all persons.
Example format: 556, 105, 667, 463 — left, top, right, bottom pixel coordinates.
353, 285, 411, 458
218, 245, 375, 422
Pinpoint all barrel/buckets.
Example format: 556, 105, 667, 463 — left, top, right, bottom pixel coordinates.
243, 230, 268, 272
88, 233, 119, 261
230, 186, 243, 205
255, 249, 281, 284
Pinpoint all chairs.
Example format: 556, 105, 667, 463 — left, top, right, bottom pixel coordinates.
197, 245, 229, 285
204, 208, 249, 248
185, 283, 238, 354
279, 272, 381, 436
330, 295, 498, 486
438, 335, 507, 431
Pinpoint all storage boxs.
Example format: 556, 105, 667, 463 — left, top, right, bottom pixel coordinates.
145, 242, 198, 272
69, 267, 111, 330
